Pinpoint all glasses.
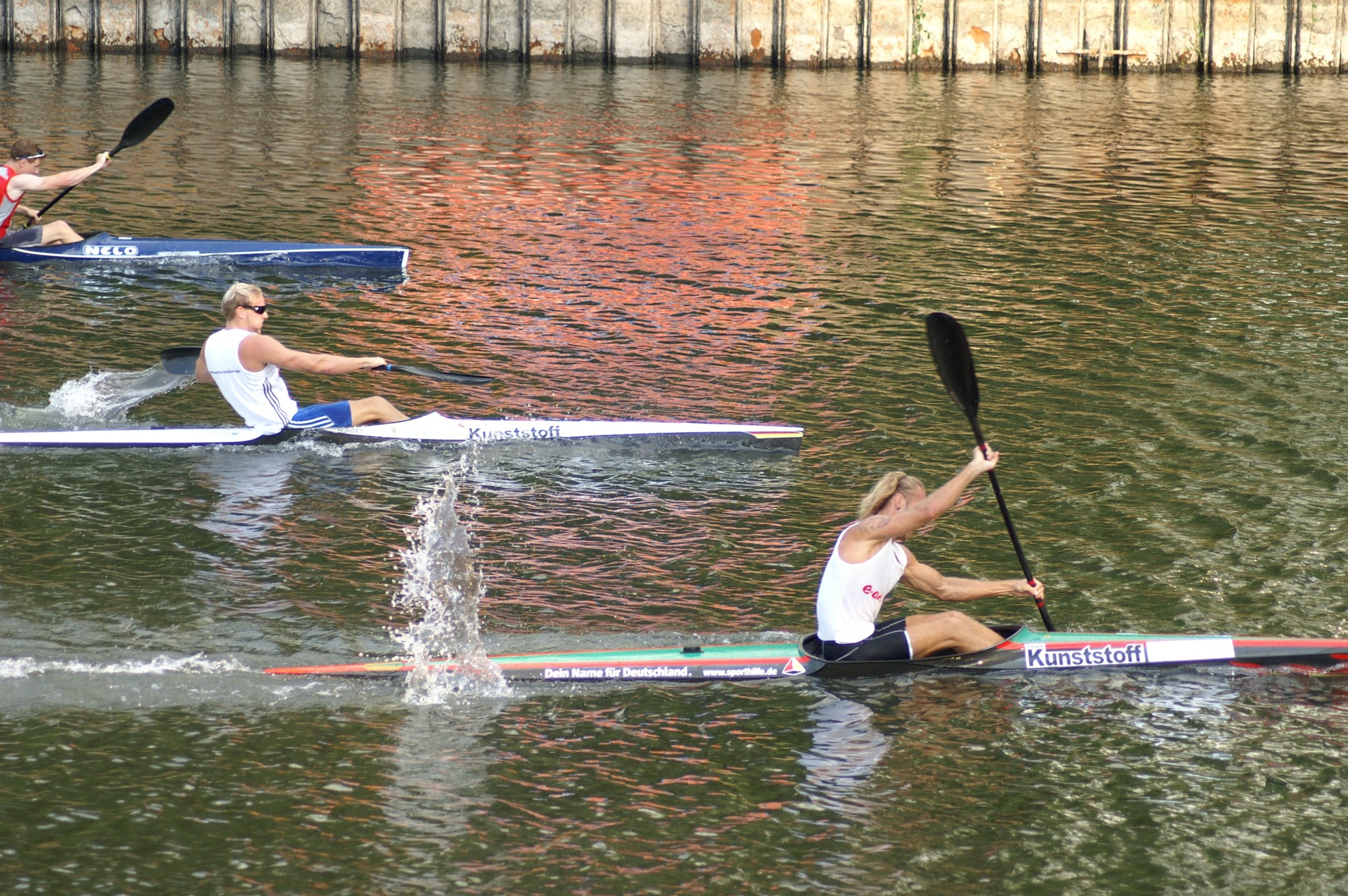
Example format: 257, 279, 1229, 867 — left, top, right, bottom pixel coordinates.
236, 304, 266, 314
15, 148, 44, 160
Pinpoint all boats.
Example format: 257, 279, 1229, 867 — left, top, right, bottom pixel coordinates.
0, 231, 410, 268
265, 624, 1348, 681
0, 410, 803, 455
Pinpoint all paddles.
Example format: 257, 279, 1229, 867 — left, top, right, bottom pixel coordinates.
923, 311, 1057, 637
19, 94, 176, 230
158, 345, 499, 386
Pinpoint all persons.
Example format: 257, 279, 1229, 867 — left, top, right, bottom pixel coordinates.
817, 443, 1044, 662
195, 282, 411, 429
0, 138, 113, 249
